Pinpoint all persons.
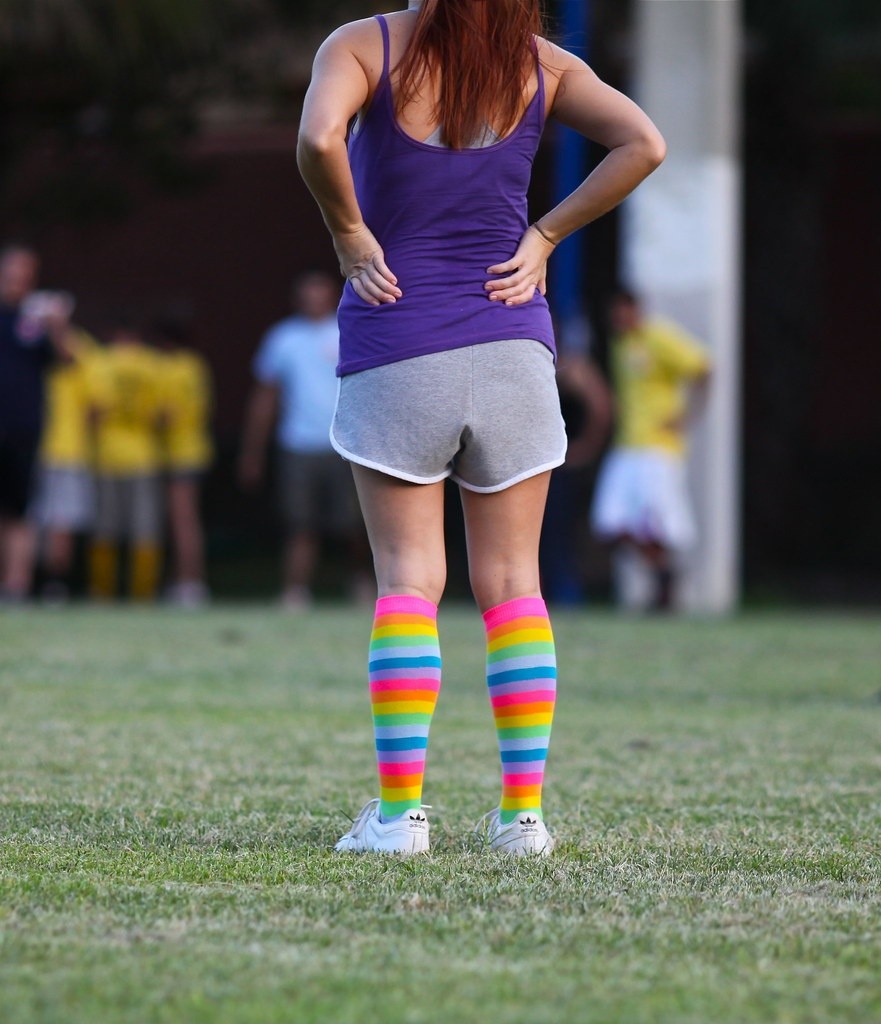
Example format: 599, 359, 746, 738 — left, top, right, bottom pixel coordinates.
297, 0, 665, 858
0, 242, 711, 614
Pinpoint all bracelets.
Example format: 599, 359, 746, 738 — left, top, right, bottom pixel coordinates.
534, 223, 556, 245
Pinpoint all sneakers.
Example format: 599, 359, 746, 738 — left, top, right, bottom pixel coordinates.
474, 806, 553, 860
333, 797, 430, 855
